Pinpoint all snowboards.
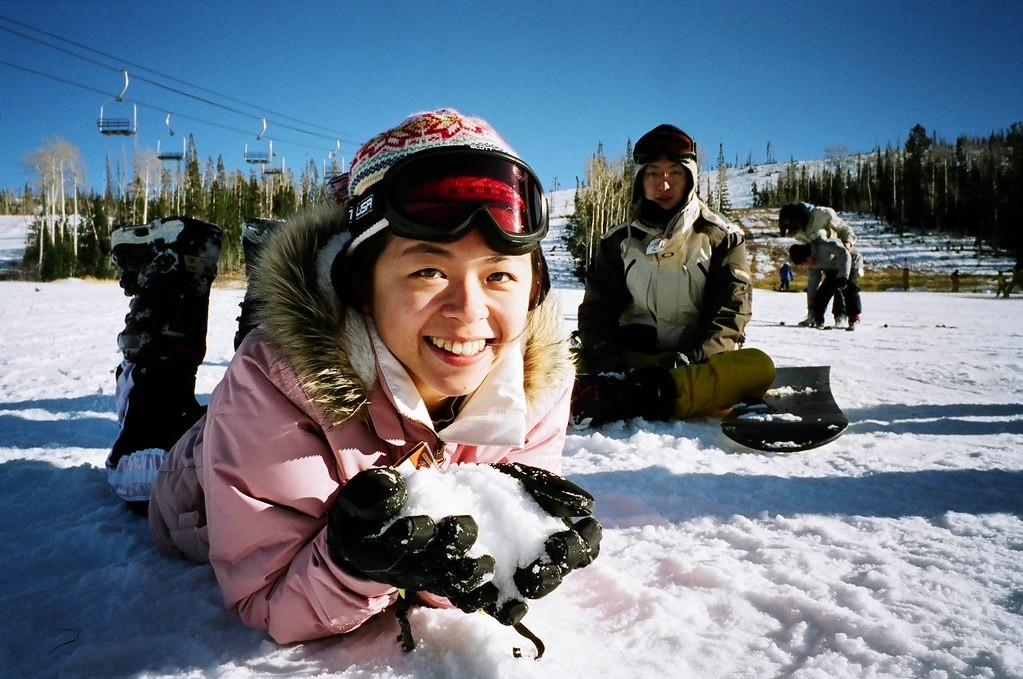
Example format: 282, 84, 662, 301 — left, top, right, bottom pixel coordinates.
722, 363, 849, 453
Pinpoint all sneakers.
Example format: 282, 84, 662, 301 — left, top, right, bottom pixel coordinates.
798, 317, 824, 328
845, 320, 859, 331
568, 373, 673, 427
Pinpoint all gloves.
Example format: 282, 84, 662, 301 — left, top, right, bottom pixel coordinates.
481, 462, 604, 625
326, 468, 500, 614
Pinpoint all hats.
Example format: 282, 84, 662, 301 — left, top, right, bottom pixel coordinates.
347, 109, 528, 235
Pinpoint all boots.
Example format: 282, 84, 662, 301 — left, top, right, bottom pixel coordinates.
233, 218, 293, 353
110, 217, 223, 365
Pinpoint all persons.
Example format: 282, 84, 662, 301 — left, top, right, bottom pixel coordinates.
778, 201, 864, 331
951, 269, 960, 292
103, 109, 603, 646
992, 258, 1023, 299
562, 123, 777, 429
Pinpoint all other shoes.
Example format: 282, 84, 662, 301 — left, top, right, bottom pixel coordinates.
833, 315, 848, 329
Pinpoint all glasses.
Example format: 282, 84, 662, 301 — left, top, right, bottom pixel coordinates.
382, 145, 548, 256
632, 130, 697, 164
